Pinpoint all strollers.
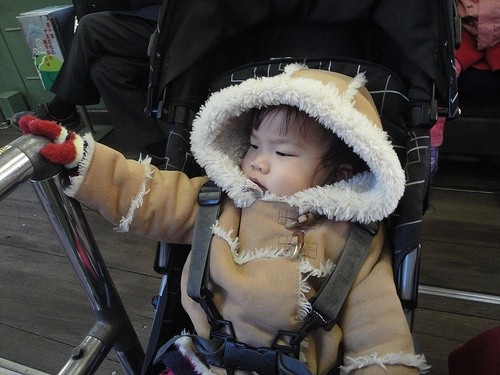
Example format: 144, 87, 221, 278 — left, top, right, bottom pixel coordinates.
132, 1, 462, 375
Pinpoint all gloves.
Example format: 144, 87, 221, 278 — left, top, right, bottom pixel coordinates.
18, 113, 88, 178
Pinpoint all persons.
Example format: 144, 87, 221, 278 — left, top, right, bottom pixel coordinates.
18, 58, 438, 375
427, 0, 500, 178
10, 0, 179, 173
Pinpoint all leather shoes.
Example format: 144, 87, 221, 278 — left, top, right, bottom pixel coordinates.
10, 102, 81, 133
